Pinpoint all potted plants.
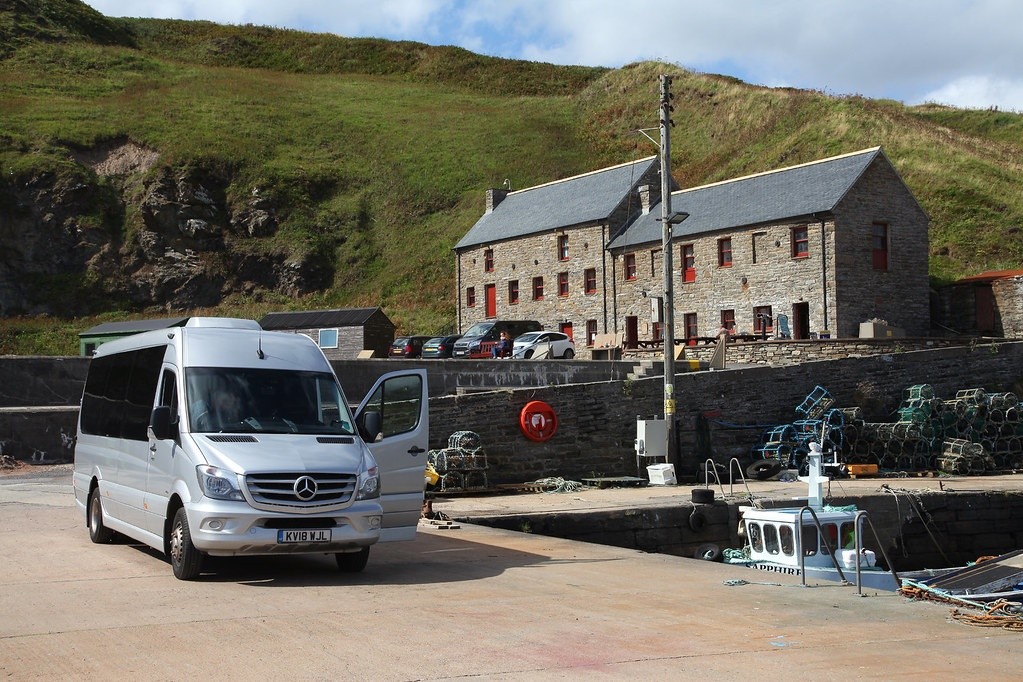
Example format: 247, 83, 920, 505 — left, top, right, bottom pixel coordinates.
606, 343, 609, 348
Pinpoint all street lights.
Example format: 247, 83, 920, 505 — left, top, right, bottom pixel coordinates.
661, 210, 691, 484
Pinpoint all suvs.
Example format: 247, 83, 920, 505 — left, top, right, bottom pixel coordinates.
388, 334, 435, 359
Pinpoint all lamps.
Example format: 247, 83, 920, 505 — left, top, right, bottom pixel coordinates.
503, 178, 513, 190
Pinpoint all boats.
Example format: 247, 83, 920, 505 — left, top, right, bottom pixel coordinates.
900, 548, 1023, 604
703, 439, 963, 592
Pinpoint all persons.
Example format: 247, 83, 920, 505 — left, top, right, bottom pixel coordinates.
491, 331, 511, 359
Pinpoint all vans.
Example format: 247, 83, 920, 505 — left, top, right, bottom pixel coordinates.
452, 318, 545, 359
71, 316, 429, 581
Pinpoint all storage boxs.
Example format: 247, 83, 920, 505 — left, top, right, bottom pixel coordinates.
645, 462, 678, 485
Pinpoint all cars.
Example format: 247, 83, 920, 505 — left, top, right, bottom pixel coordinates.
420, 335, 463, 359
497, 331, 576, 359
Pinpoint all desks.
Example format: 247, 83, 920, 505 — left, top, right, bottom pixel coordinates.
587, 347, 621, 360
858, 323, 905, 337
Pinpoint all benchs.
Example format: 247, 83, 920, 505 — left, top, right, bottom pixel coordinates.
470, 340, 512, 358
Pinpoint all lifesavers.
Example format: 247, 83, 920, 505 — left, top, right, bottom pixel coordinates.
690, 512, 708, 532
695, 461, 736, 484
746, 458, 782, 480
693, 543, 726, 562
